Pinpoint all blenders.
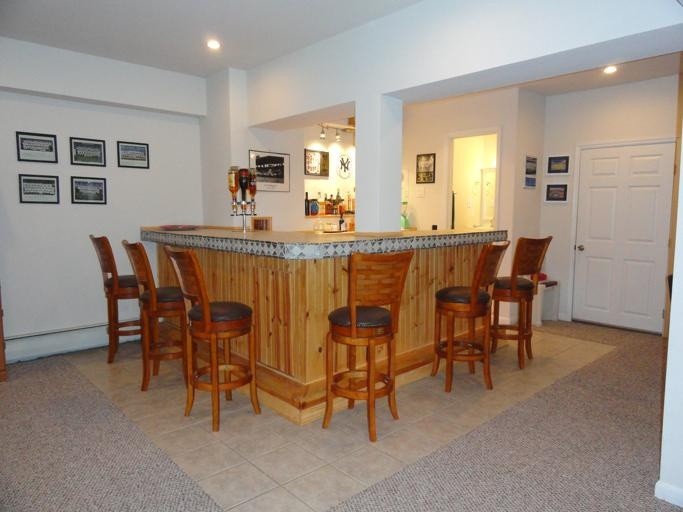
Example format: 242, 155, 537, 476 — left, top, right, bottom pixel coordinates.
401, 201, 411, 228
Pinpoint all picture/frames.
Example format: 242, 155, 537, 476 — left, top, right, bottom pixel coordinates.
522, 154, 573, 204
117, 141, 149, 169
70, 136, 106, 166
71, 176, 106, 204
19, 173, 59, 204
16, 131, 58, 163
248, 150, 291, 192
252, 216, 273, 232
415, 153, 436, 184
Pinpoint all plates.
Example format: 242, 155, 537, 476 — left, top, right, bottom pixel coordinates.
336, 154, 353, 179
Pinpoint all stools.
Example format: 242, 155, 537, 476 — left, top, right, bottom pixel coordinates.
532, 282, 560, 326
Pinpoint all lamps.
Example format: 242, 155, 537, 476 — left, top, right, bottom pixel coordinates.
336, 126, 343, 143
318, 122, 330, 144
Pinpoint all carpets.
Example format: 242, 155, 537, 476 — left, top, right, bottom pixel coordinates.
1, 317, 681, 512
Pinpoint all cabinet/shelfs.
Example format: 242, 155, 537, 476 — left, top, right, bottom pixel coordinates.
308, 214, 354, 234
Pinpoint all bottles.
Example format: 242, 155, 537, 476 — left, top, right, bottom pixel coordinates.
305, 187, 352, 217
339, 212, 344, 231
227, 166, 257, 211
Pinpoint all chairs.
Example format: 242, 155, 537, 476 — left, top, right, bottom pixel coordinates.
163, 245, 262, 433
121, 240, 199, 392
485, 235, 555, 369
89, 234, 141, 364
431, 239, 511, 393
322, 248, 416, 442
